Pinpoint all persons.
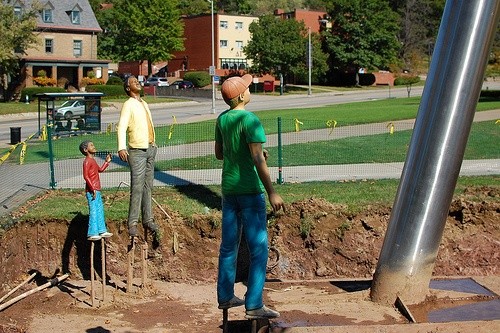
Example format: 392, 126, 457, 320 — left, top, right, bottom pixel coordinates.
79, 141, 114, 241
214, 74, 286, 319
118, 75, 160, 236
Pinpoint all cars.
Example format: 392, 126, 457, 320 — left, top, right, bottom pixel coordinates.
50, 99, 102, 121
170, 80, 195, 91
110, 71, 131, 82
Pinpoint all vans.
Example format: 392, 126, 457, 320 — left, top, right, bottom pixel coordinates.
144, 77, 169, 88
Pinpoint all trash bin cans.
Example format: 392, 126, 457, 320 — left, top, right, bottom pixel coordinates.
141, 82, 159, 97
10, 127, 22, 144
